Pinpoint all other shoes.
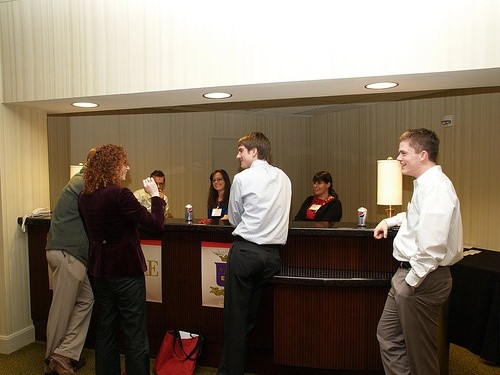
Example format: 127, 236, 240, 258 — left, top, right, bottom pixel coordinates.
44, 354, 74, 373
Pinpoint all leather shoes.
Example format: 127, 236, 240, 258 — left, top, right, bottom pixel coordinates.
398, 261, 411, 269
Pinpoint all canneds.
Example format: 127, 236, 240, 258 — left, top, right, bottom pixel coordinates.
357, 207, 368, 227
185, 204, 193, 221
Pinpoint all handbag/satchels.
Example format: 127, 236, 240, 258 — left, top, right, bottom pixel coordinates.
153, 328, 200, 374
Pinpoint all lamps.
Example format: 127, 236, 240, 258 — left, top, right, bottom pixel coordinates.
376, 156, 403, 219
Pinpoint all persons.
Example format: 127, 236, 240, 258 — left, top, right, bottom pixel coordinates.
215, 132, 292, 375
373, 128, 463, 375
133, 170, 169, 218
77, 144, 166, 375
295, 171, 342, 222
45, 149, 96, 375
207, 169, 236, 219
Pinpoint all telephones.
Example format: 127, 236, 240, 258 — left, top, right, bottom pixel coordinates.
21, 207, 51, 232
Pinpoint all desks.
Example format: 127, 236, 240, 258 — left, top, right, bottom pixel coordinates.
444, 248, 500, 366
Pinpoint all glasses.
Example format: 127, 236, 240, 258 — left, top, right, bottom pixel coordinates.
213, 178, 222, 181
